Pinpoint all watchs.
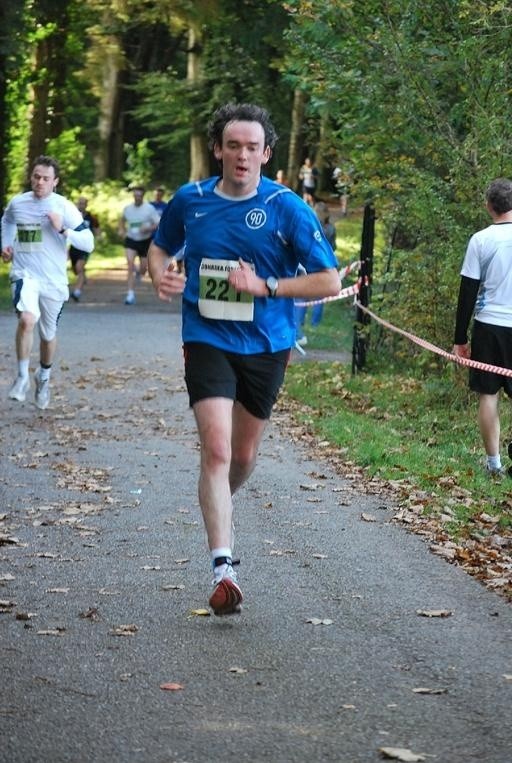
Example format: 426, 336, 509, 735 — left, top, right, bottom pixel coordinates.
58, 225, 66, 233
266, 276, 278, 299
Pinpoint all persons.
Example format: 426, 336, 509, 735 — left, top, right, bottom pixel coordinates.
147, 102, 341, 617
0, 155, 95, 411
117, 188, 160, 305
69, 198, 100, 302
148, 188, 168, 217
452, 176, 512, 476
168, 246, 185, 272
273, 158, 354, 328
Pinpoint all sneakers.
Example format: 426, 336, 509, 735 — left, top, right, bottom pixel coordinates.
72, 289, 81, 299
35, 367, 49, 409
8, 376, 30, 401
209, 577, 242, 617
125, 292, 136, 304
